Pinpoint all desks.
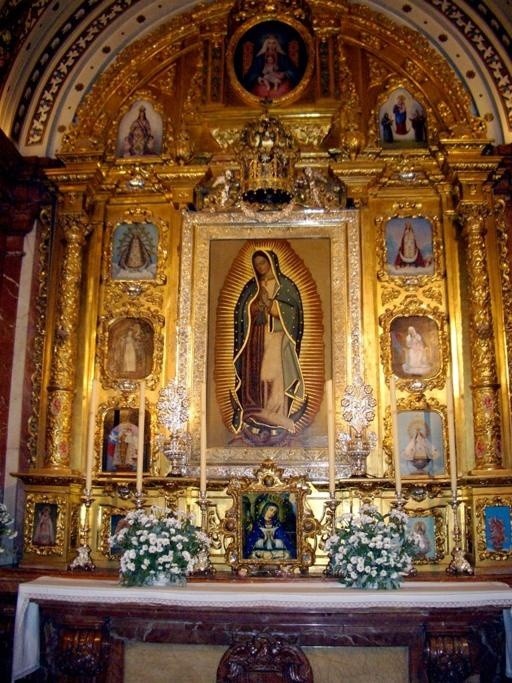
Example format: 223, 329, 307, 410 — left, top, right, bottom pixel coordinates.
23, 576, 510, 683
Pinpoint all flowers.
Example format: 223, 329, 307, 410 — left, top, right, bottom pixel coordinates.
107, 503, 207, 585
321, 499, 416, 591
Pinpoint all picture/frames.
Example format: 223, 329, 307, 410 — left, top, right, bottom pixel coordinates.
372, 201, 456, 479
21, 492, 68, 555
370, 75, 435, 156
222, 458, 318, 576
226, 12, 314, 110
90, 203, 175, 484
108, 87, 175, 164
178, 210, 364, 483
382, 494, 509, 578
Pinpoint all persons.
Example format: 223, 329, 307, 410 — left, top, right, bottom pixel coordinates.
34, 509, 55, 546
382, 93, 426, 142
400, 221, 419, 266
108, 410, 141, 471
122, 105, 155, 157
402, 326, 431, 375
230, 248, 306, 434
117, 329, 139, 374
249, 35, 297, 97
247, 503, 294, 561
